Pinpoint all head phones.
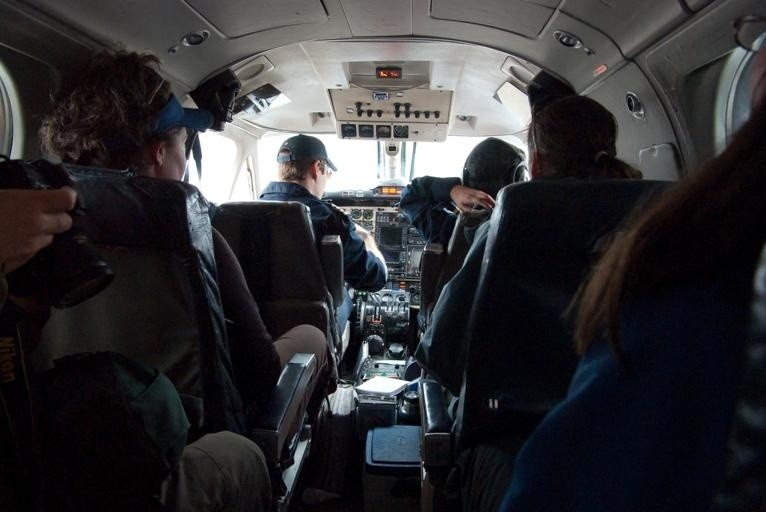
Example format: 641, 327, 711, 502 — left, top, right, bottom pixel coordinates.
487, 134, 530, 184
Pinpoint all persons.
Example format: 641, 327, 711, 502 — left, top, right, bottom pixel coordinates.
415, 95, 641, 398
1, 43, 340, 512
398, 135, 527, 245
498, 83, 766, 511
257, 132, 389, 338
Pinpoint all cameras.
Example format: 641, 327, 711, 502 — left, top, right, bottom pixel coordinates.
0, 156, 114, 312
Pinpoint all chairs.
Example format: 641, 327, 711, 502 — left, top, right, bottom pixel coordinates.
38, 174, 326, 512
417, 178, 684, 511
207, 202, 353, 398
420, 209, 491, 333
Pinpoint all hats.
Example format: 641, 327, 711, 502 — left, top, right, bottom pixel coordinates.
464, 137, 526, 184
137, 90, 214, 144
276, 134, 338, 172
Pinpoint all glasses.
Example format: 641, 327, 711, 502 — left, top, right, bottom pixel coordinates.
733, 12, 766, 53
532, 94, 565, 169
